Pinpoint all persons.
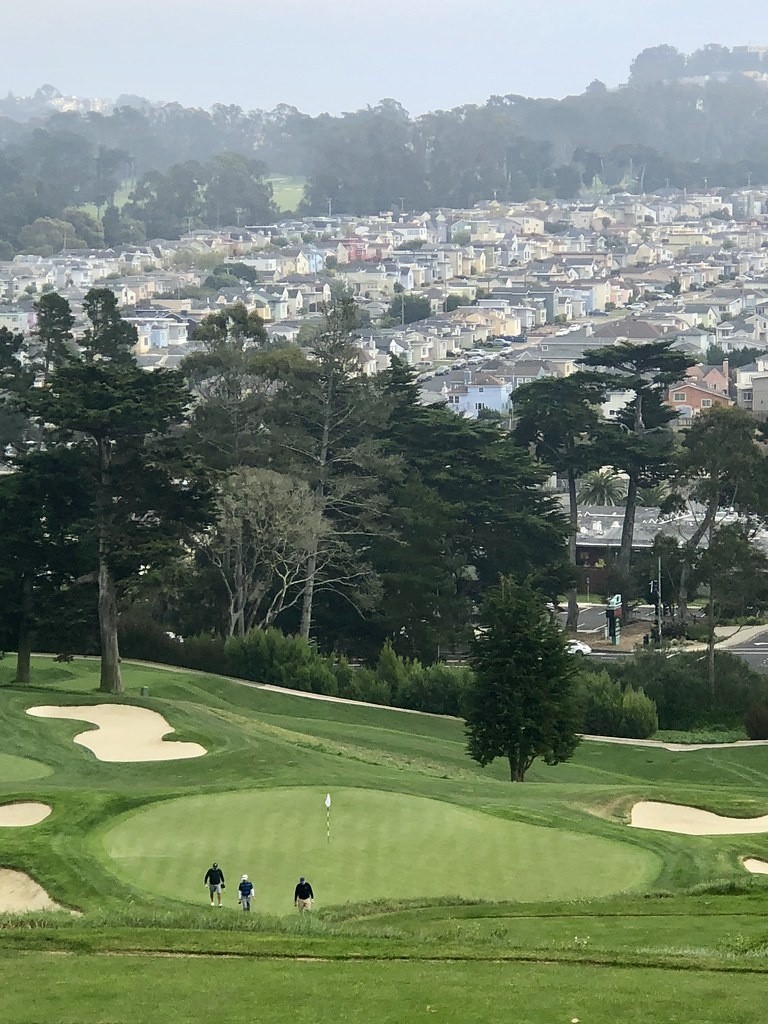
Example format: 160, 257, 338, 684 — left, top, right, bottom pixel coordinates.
204, 863, 226, 908
294, 877, 314, 917
238, 874, 255, 911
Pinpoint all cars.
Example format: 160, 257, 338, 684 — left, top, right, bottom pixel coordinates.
564, 639, 592, 657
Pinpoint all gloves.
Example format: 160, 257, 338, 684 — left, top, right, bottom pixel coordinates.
252, 897, 255, 900
312, 900, 315, 902
294, 902, 297, 907
205, 885, 208, 888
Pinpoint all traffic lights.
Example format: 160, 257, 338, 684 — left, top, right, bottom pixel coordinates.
650, 580, 658, 594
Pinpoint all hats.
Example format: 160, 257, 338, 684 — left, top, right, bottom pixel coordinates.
300, 878, 305, 881
241, 874, 248, 880
213, 863, 218, 867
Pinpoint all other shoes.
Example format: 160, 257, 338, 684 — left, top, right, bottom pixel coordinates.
211, 902, 215, 907
218, 904, 223, 907
